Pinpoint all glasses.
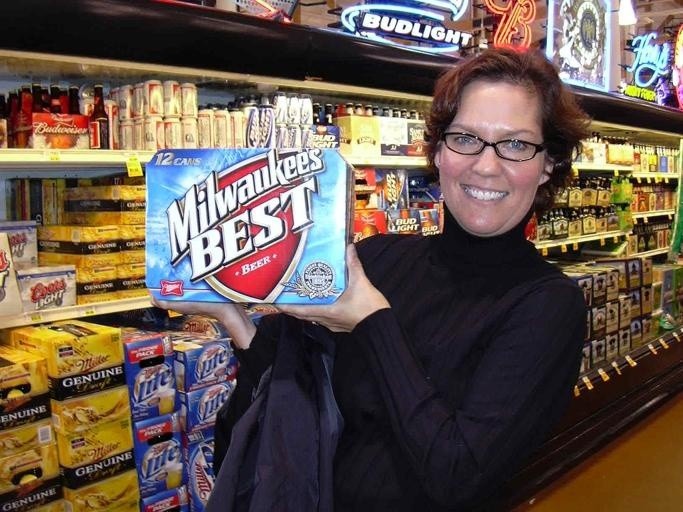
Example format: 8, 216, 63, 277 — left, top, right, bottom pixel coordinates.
440, 130, 545, 162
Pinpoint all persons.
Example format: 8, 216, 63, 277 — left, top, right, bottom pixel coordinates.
151, 46, 598, 511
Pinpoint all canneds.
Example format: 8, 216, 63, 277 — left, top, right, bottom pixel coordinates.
80, 79, 314, 151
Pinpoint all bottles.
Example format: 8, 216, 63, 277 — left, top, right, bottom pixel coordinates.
0, 83, 111, 150
538, 133, 629, 239
312, 101, 425, 127
633, 140, 680, 235
